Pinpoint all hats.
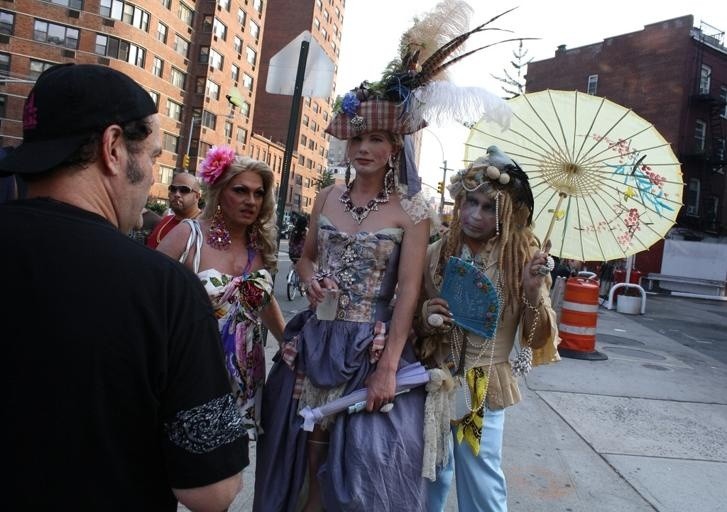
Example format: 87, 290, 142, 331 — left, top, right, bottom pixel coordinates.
3, 62, 160, 173
324, 101, 428, 140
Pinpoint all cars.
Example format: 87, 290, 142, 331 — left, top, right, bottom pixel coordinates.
280, 225, 295, 240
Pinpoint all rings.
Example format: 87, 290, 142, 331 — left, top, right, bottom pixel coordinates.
426, 312, 443, 327
378, 401, 393, 413
544, 255, 554, 271
537, 263, 548, 275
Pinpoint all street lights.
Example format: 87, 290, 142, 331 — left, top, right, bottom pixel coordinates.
184, 112, 236, 174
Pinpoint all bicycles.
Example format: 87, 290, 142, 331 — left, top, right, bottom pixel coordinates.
287, 257, 307, 302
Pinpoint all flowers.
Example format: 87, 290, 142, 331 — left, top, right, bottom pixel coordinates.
196, 142, 236, 188
331, 92, 360, 118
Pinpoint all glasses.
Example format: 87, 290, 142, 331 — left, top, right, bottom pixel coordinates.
168, 185, 198, 194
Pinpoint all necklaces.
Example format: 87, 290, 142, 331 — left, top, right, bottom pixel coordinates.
449, 258, 506, 415
156, 210, 201, 244
339, 180, 393, 225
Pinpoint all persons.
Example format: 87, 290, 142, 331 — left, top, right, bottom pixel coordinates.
288, 216, 308, 296
412, 146, 560, 510
251, 128, 427, 512
146, 173, 203, 250
157, 143, 286, 441
128, 208, 149, 239
0, 64, 248, 512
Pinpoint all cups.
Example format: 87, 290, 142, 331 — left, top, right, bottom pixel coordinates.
315, 283, 342, 323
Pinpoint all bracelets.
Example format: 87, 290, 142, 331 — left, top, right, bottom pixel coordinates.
521, 290, 544, 311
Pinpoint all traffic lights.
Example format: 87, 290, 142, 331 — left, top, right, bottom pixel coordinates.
181, 154, 189, 169
436, 181, 444, 195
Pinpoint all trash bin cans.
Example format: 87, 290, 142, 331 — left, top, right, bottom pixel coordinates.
613, 268, 642, 303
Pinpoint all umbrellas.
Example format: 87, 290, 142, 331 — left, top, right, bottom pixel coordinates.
463, 88, 687, 264
